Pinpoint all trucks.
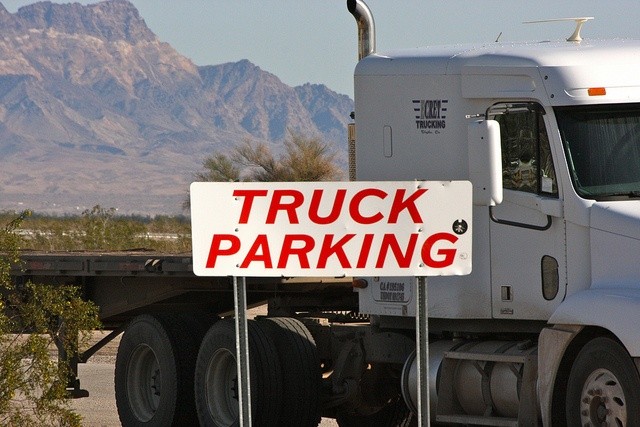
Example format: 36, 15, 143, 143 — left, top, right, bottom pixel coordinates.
0, 0, 639, 426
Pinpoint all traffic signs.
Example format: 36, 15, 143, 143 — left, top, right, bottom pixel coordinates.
189, 181, 473, 278
